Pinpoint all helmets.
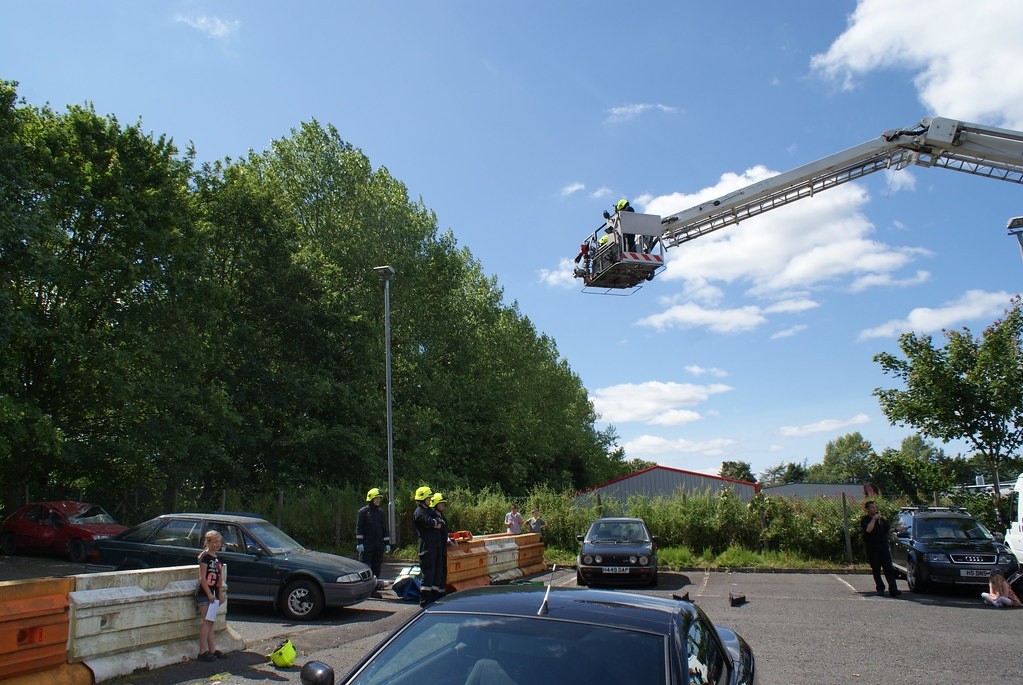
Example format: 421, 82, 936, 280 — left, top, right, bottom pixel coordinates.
600, 235, 608, 244
366, 488, 383, 501
616, 199, 628, 211
429, 493, 446, 507
415, 487, 433, 501
269, 640, 298, 667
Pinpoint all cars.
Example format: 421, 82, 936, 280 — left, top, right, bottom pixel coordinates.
300, 585, 755, 685
0, 500, 130, 563
94, 512, 377, 622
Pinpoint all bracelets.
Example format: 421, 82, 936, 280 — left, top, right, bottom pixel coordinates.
895, 528, 897, 532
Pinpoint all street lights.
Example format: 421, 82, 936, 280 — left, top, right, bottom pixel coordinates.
372, 263, 396, 550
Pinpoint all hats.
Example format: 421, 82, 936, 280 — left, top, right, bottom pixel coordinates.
990, 569, 1003, 577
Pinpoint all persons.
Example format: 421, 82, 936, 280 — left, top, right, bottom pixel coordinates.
981, 568, 1021, 609
413, 486, 449, 608
194, 530, 227, 662
505, 504, 524, 533
860, 501, 905, 596
524, 508, 548, 542
356, 488, 390, 599
617, 199, 636, 252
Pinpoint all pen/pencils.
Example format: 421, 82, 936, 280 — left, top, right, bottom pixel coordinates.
214, 590, 215, 596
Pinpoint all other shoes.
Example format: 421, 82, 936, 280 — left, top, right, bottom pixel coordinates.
984, 600, 992, 607
209, 650, 226, 659
998, 603, 1003, 608
198, 651, 215, 661
877, 588, 885, 596
889, 589, 901, 596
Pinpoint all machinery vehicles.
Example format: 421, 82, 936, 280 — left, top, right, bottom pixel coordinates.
571, 116, 1023, 296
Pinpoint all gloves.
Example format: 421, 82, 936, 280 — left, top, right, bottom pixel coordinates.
356, 544, 364, 555
386, 545, 390, 552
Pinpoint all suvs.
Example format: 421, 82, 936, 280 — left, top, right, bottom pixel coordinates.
576, 514, 660, 586
887, 506, 1021, 594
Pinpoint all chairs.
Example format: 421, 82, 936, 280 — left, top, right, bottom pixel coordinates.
216, 524, 234, 553
918, 522, 937, 538
623, 529, 638, 539
599, 531, 612, 538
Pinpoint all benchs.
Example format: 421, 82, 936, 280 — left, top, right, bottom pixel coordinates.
154, 536, 194, 548
